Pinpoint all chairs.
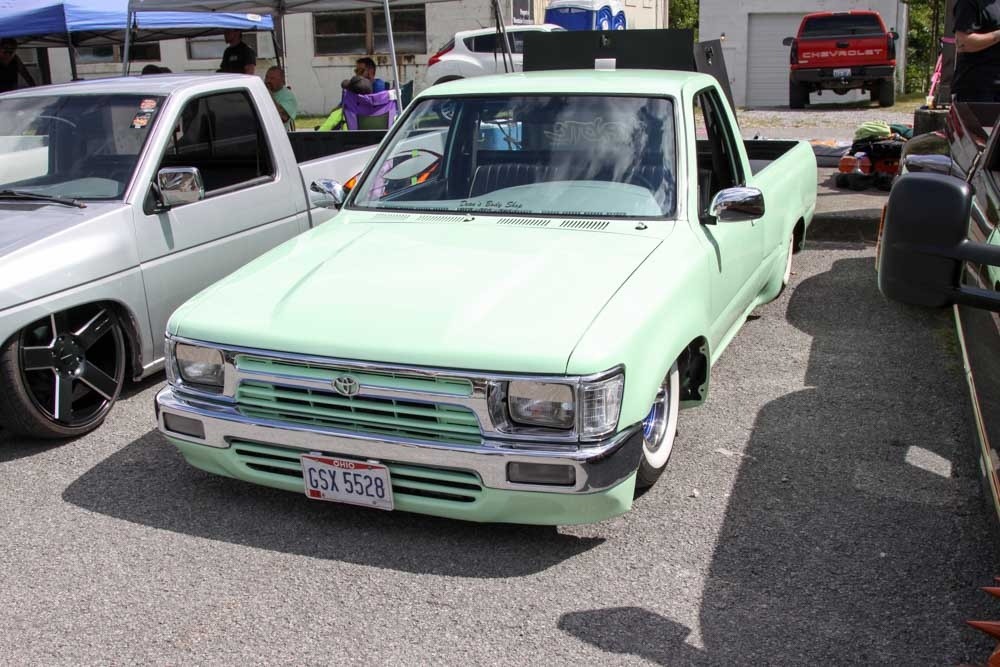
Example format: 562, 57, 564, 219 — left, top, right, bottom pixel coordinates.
331, 79, 414, 131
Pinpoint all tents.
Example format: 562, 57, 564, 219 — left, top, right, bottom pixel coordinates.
122, 0, 516, 117
0, 0, 283, 80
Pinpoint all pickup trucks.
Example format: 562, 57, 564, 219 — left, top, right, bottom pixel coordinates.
783, 8, 917, 117
156, 67, 822, 547
0, 74, 460, 447
869, 93, 1000, 330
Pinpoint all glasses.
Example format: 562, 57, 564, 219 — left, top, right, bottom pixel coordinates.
354, 66, 368, 74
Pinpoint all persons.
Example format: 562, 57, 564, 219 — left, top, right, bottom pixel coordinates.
141, 64, 170, 75
264, 65, 297, 132
216, 28, 257, 75
334, 56, 385, 109
952, 0, 1000, 102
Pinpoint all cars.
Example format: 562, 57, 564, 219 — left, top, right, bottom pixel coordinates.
421, 23, 573, 131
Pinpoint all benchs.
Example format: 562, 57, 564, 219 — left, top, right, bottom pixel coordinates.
469, 162, 672, 212
